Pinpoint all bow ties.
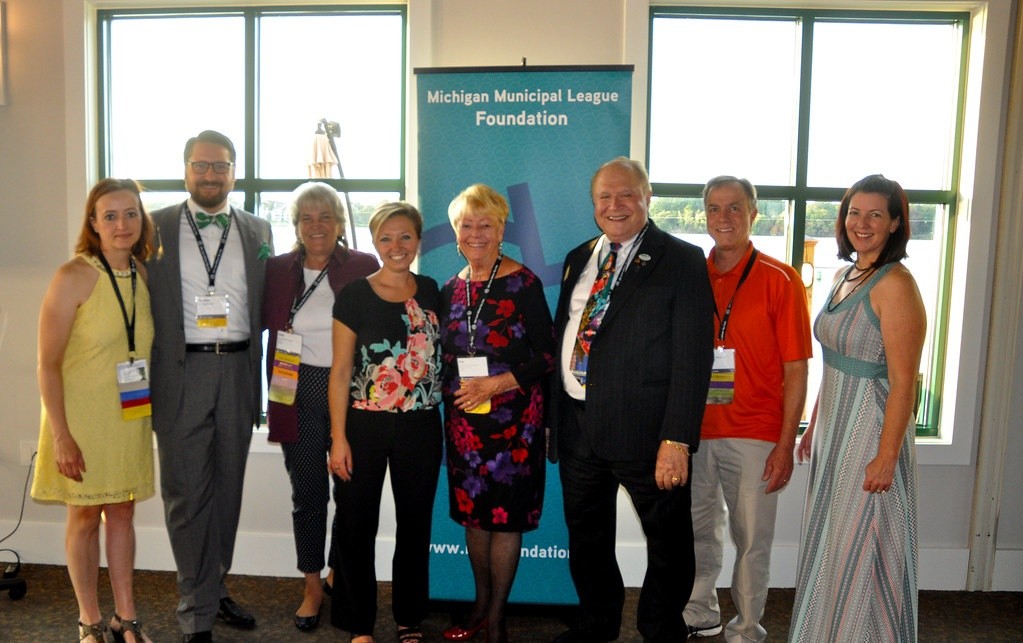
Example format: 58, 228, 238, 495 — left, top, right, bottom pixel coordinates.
195, 211, 229, 231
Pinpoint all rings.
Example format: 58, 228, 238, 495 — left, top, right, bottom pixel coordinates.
467, 401, 472, 406
876, 489, 882, 494
335, 467, 340, 471
783, 479, 789, 484
671, 477, 680, 480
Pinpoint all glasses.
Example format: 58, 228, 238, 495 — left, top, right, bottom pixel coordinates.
186, 160, 234, 174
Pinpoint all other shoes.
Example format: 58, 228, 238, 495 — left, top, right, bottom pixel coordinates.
685, 623, 723, 639
551, 630, 607, 643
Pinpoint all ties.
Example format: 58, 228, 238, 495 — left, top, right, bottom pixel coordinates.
569, 241, 623, 388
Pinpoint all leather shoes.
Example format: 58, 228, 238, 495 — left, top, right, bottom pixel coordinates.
215, 597, 255, 629
322, 580, 332, 596
183, 630, 213, 643
443, 618, 488, 641
483, 623, 488, 643
294, 602, 323, 632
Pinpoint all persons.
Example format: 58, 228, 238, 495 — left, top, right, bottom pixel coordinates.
261, 180, 382, 633
327, 200, 444, 643
439, 183, 553, 643
144, 130, 274, 643
682, 175, 814, 643
544, 155, 714, 643
29, 178, 157, 643
795, 174, 928, 643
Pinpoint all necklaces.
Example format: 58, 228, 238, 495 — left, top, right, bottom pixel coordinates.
89, 253, 137, 277
827, 261, 877, 312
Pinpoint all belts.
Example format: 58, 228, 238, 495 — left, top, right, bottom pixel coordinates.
185, 338, 250, 355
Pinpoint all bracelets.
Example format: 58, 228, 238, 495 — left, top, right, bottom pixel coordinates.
665, 440, 689, 455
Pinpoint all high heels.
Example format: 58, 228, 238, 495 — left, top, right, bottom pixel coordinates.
78, 618, 108, 643
109, 613, 146, 643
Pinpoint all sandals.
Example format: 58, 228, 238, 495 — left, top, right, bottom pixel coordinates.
350, 632, 374, 643
396, 623, 424, 643
